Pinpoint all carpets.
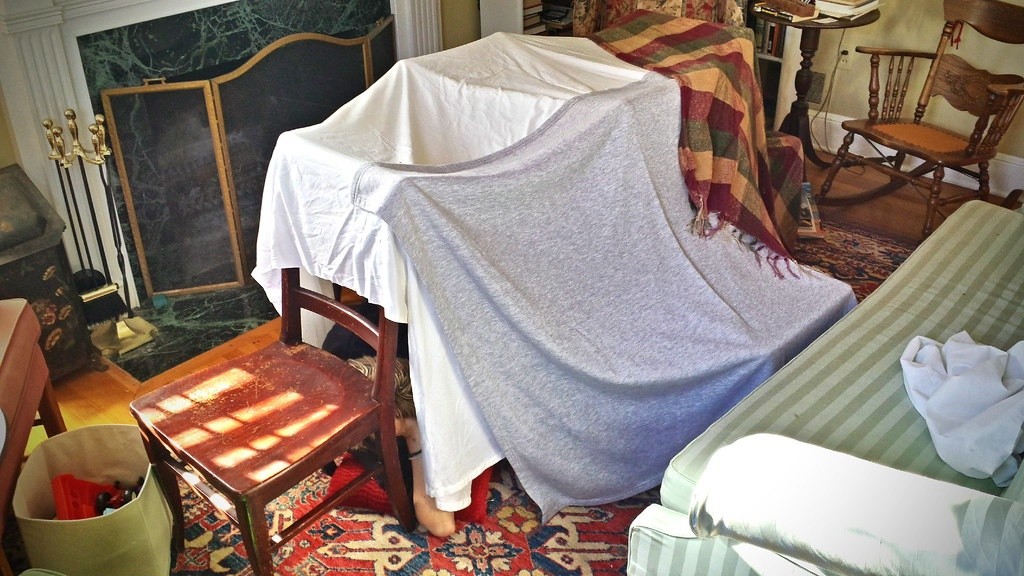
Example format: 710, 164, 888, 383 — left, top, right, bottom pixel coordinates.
169, 207, 922, 576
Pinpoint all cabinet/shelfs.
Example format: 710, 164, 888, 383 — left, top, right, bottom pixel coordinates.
0, 162, 109, 381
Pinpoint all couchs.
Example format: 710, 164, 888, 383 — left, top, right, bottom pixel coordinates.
627, 199, 1023, 576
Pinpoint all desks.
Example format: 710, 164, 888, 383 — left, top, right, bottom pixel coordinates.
749, 3, 880, 182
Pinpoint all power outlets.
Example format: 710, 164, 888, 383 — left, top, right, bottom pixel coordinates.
837, 47, 854, 70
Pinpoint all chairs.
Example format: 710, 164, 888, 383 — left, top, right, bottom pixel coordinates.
128, 133, 412, 576
811, 0, 1024, 246
